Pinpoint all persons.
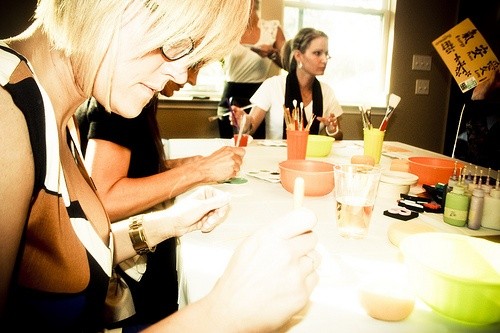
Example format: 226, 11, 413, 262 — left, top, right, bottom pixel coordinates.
0, 0, 344, 333
468, 69, 500, 172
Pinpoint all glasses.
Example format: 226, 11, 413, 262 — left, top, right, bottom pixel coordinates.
145, 0, 203, 69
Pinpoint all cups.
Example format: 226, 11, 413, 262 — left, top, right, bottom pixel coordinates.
234, 114, 250, 147
286, 129, 310, 160
365, 245, 421, 322
334, 164, 383, 241
362, 128, 386, 167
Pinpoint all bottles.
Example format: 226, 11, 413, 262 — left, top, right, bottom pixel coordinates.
442, 160, 500, 231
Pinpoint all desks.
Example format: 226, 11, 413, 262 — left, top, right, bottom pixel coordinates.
169, 138, 500, 333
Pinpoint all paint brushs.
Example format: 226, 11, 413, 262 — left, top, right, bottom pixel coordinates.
286, 229, 313, 242
380, 93, 401, 132
359, 105, 372, 128
208, 103, 257, 123
236, 114, 246, 148
283, 99, 316, 131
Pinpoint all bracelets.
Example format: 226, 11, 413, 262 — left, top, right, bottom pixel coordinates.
325, 124, 339, 136
128, 213, 157, 254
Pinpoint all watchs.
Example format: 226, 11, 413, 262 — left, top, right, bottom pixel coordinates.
269, 48, 278, 61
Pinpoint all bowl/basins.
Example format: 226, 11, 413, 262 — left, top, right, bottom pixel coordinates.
407, 156, 465, 187
306, 135, 335, 158
399, 231, 500, 326
278, 159, 340, 197
374, 171, 420, 210
